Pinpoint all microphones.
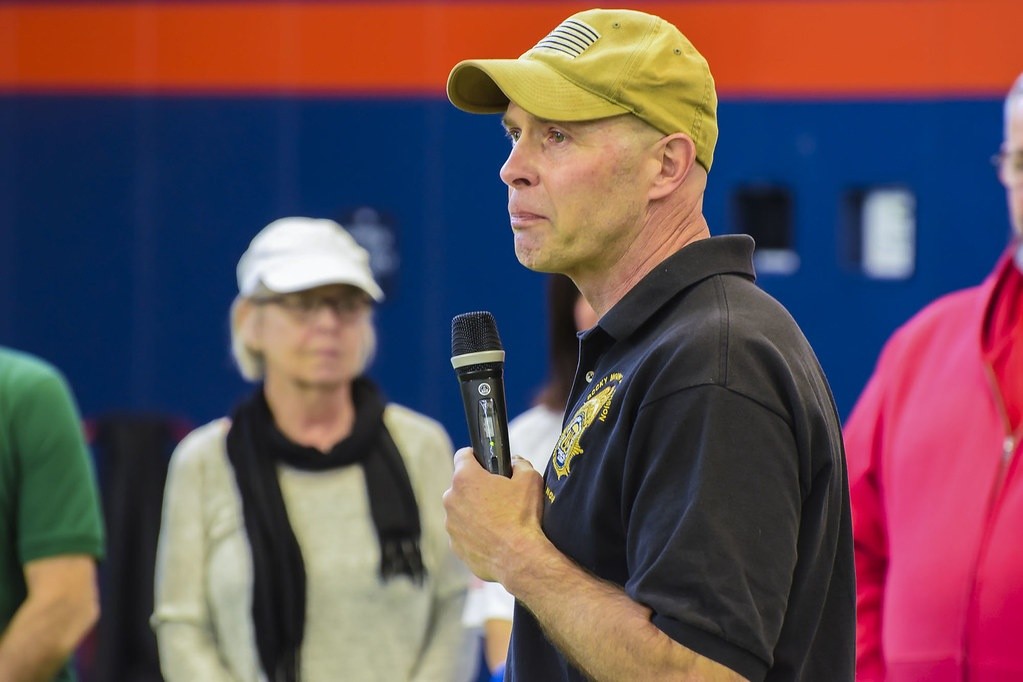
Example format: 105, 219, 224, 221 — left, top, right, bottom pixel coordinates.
449, 312, 514, 480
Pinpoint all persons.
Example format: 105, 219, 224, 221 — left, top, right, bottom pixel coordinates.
839, 72, 1023, 682
463, 268, 602, 678
151, 218, 483, 682
436, 6, 860, 682
0, 346, 105, 682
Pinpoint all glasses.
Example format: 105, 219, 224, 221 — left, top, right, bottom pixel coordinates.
250, 293, 368, 318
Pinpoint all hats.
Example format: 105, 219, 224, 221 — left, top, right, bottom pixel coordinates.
236, 216, 383, 301
445, 8, 720, 174
